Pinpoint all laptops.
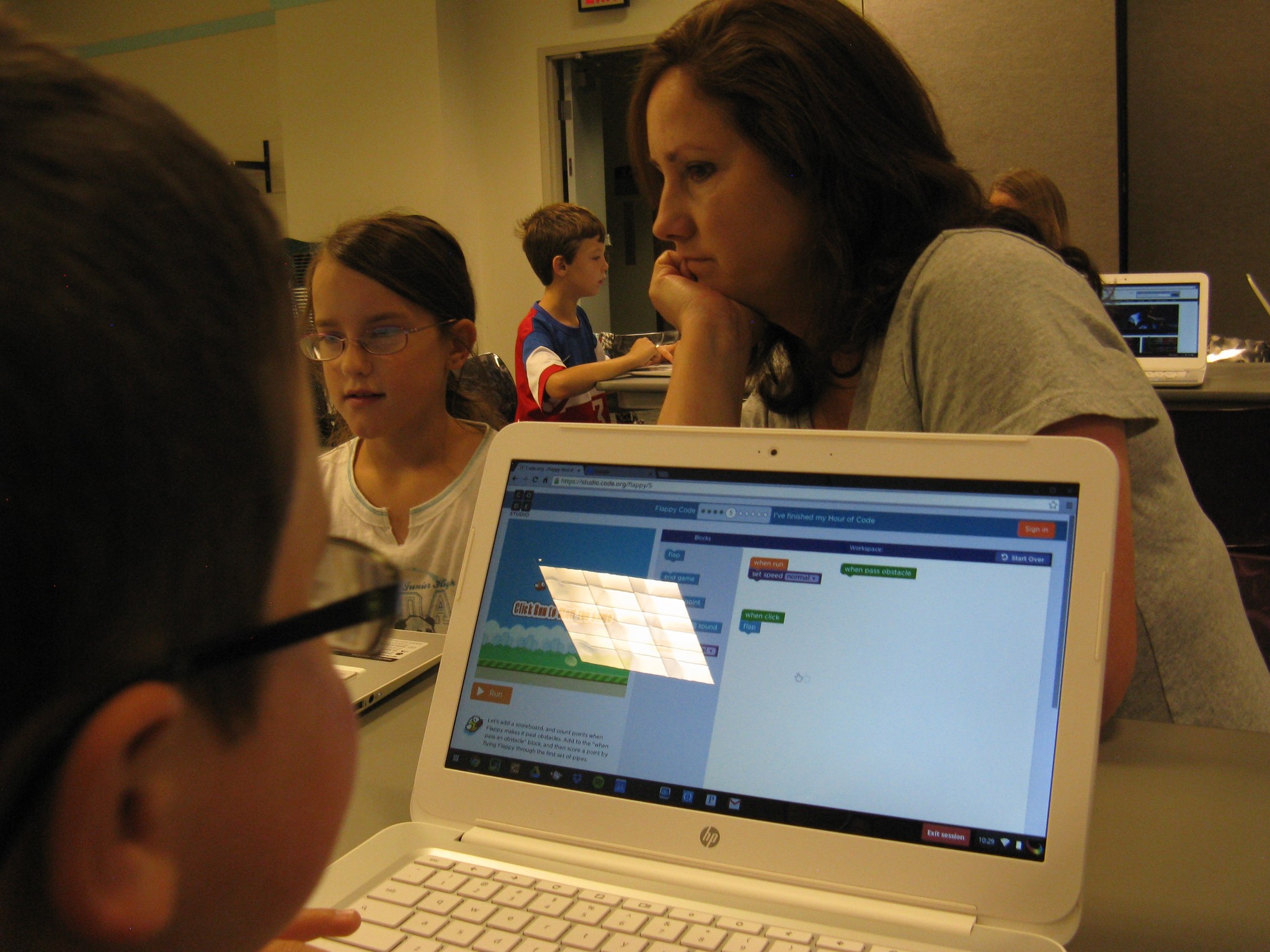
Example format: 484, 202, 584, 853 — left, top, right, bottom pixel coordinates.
303, 419, 1120, 952
1098, 272, 1209, 386
317, 623, 447, 713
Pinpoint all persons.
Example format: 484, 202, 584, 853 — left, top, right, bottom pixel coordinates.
295, 212, 508, 634
626, 1, 1269, 732
0, 19, 406, 952
988, 168, 1073, 250
515, 202, 678, 423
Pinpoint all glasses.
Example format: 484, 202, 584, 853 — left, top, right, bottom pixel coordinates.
12, 535, 403, 842
297, 318, 459, 361
